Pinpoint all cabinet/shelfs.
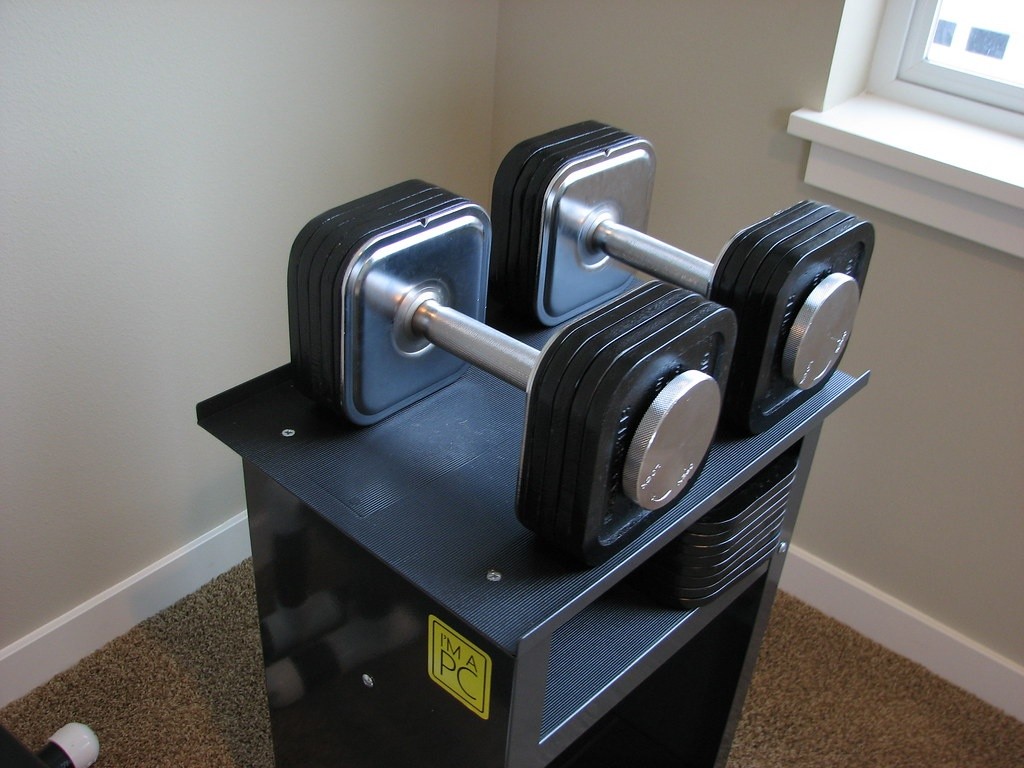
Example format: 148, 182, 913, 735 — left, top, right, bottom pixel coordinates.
196, 277, 871, 768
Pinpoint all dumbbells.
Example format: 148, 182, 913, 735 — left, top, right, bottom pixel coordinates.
290, 178, 738, 569
488, 118, 877, 437
